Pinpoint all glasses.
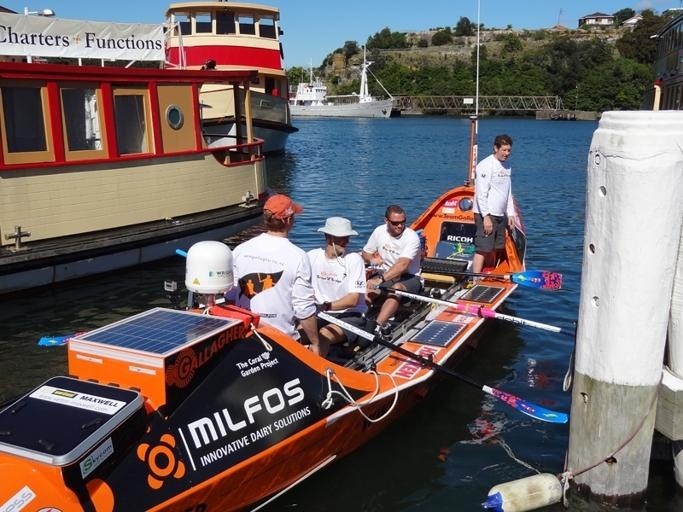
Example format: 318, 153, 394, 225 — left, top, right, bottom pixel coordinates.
385, 216, 406, 225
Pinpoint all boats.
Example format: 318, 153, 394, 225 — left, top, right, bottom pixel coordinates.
0, 7, 269, 298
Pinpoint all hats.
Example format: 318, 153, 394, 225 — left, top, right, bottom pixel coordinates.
263, 194, 303, 219
316, 217, 359, 238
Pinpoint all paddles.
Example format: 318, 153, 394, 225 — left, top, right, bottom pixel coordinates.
419, 267, 563, 293
317, 307, 569, 428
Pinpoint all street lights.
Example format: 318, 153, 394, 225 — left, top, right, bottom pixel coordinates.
23, 6, 55, 63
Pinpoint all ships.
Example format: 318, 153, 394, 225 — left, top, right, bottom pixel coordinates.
0, 61, 274, 295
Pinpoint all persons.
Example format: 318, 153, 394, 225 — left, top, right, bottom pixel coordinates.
470, 135, 516, 284
305, 217, 369, 359
232, 194, 321, 358
361, 205, 423, 334
